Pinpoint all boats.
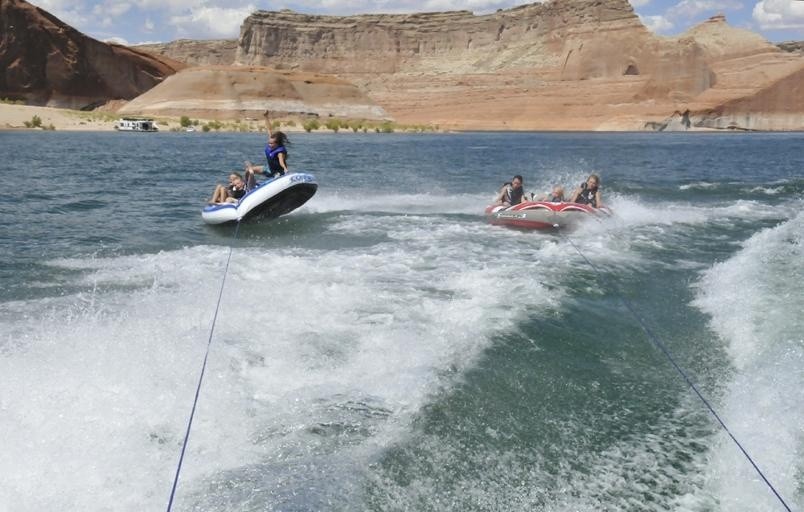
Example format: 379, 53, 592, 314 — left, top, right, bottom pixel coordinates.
199, 169, 318, 224
115, 118, 160, 133
483, 199, 613, 229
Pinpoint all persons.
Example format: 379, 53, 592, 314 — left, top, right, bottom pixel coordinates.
207, 108, 292, 204
497, 174, 602, 209
